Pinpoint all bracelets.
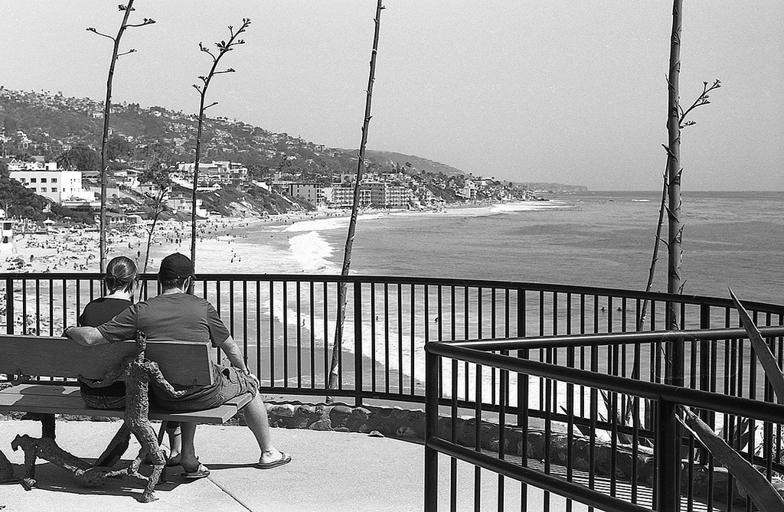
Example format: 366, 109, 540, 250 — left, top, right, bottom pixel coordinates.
246, 369, 251, 375
64, 325, 75, 335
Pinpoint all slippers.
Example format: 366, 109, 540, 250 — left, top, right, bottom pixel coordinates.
181, 463, 210, 478
256, 451, 290, 468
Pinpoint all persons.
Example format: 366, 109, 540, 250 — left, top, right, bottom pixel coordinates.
60, 253, 294, 480
78, 257, 182, 466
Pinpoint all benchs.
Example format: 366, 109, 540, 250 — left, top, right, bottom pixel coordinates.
0, 334, 254, 483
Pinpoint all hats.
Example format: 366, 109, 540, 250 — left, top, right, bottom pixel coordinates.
160, 252, 197, 280
